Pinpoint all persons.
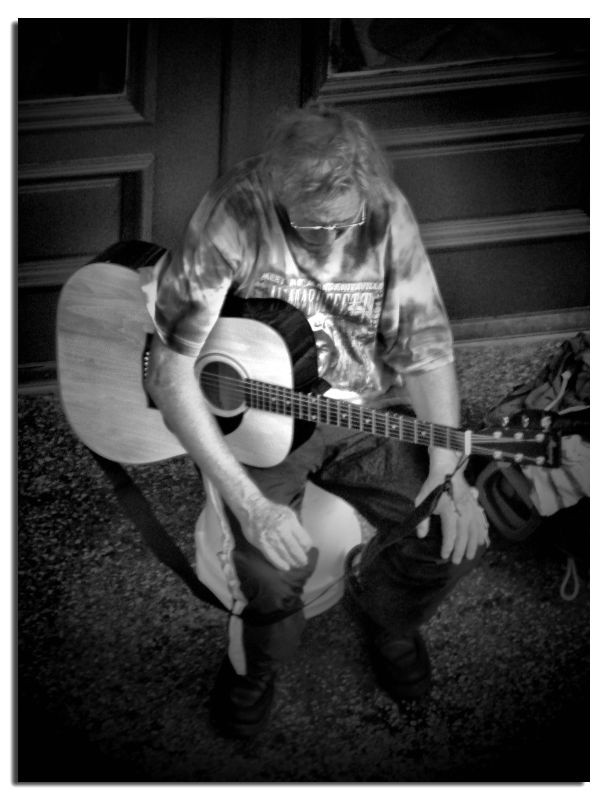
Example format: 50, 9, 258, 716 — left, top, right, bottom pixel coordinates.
146, 102, 494, 733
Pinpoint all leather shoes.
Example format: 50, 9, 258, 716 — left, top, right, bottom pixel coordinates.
209, 663, 276, 735
342, 543, 430, 698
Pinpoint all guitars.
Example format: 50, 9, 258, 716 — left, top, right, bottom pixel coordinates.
54, 240, 565, 469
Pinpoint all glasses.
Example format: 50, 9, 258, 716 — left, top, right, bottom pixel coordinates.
282, 200, 365, 232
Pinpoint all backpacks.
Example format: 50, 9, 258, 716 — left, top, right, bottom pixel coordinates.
465, 327, 589, 565
